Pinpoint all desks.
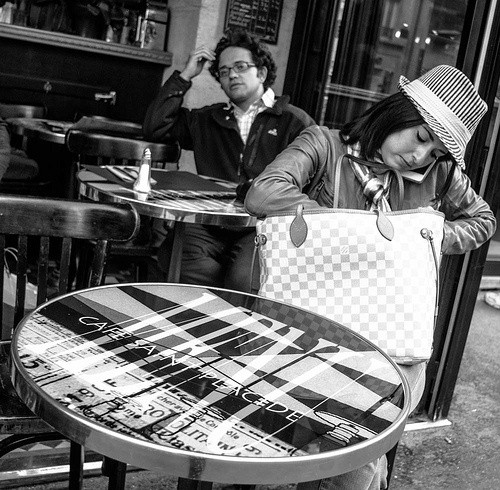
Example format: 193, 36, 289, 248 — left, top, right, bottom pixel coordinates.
9, 282, 412, 490
5, 117, 181, 200
70, 165, 258, 292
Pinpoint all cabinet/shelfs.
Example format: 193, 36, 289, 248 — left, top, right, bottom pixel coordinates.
0, 22, 173, 123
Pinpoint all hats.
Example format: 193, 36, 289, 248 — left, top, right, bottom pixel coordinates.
397, 65, 488, 171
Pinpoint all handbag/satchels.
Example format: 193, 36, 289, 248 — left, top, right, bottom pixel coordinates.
255, 155, 445, 366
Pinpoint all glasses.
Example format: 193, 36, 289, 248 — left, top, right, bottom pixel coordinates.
215, 61, 256, 80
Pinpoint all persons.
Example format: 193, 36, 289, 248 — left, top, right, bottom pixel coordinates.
142, 29, 317, 295
212, 64, 497, 490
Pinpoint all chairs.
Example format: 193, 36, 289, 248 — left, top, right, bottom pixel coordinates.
0, 102, 185, 490
